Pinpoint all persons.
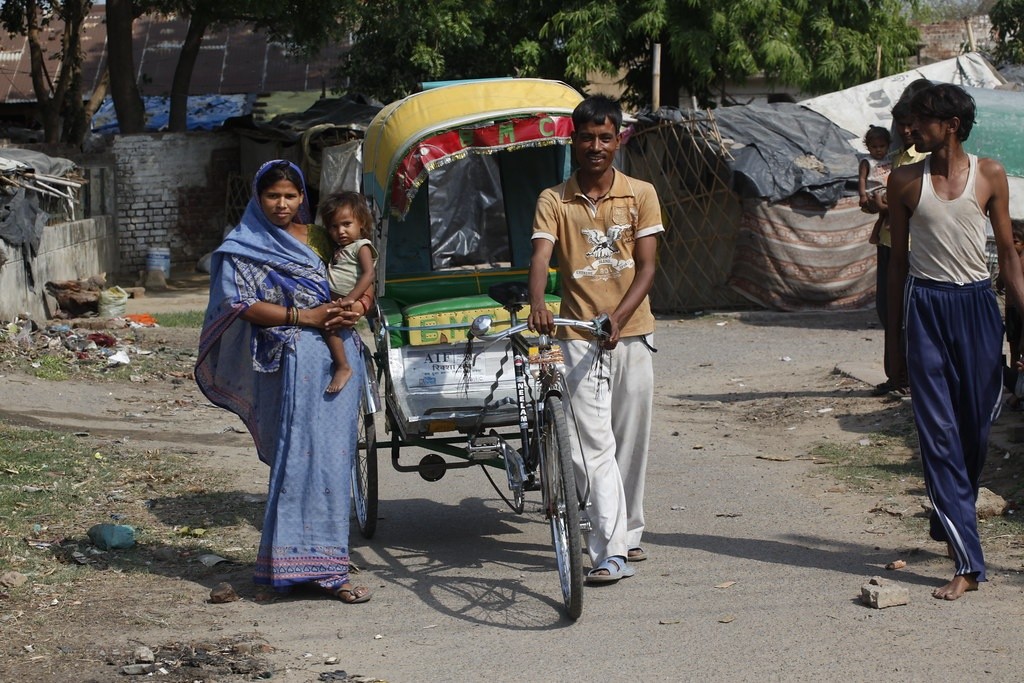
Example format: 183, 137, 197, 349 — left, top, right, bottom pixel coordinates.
887, 82, 1024, 600
997, 220, 1024, 410
528, 94, 665, 580
860, 126, 891, 244
863, 104, 931, 398
195, 160, 373, 603
325, 190, 379, 393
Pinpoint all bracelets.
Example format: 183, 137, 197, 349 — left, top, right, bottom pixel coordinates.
359, 294, 373, 315
286, 306, 300, 325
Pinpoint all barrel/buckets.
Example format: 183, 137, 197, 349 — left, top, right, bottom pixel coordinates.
144, 247, 170, 280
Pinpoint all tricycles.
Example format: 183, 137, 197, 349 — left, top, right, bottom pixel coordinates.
350, 75, 613, 619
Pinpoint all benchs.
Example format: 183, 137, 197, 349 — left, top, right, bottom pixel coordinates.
379, 266, 563, 349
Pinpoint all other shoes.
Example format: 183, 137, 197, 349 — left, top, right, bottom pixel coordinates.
889, 387, 912, 396
877, 379, 909, 391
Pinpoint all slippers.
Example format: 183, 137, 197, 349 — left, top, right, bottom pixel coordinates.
323, 582, 370, 604
586, 556, 635, 581
628, 548, 647, 560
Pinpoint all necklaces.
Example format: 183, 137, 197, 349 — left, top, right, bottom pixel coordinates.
581, 169, 615, 204
